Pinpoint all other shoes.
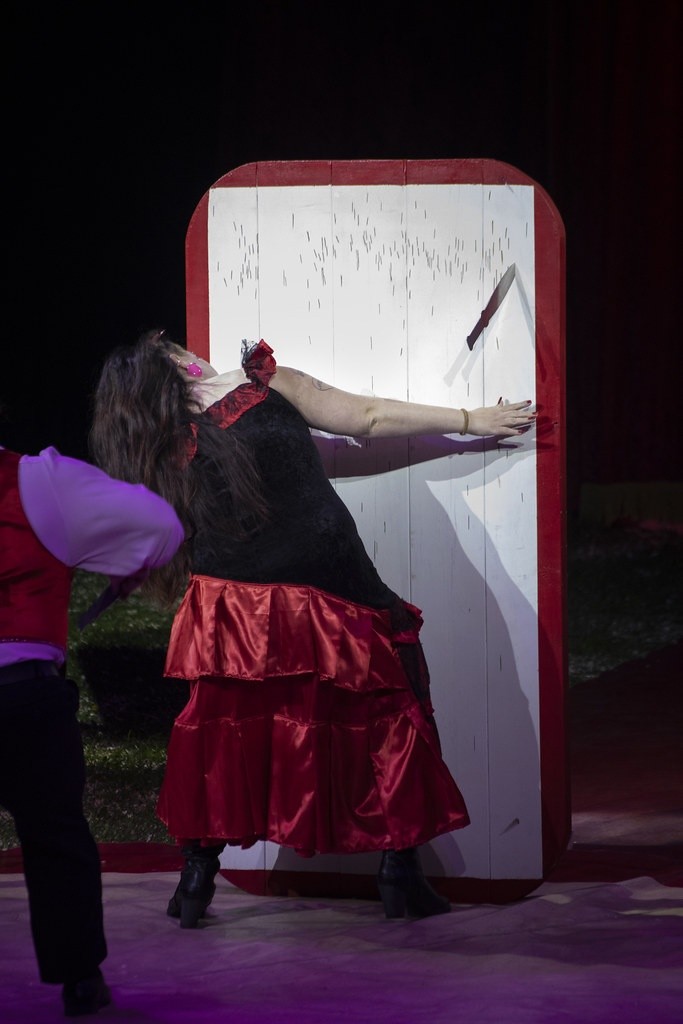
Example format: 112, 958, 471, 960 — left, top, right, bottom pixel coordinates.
61, 973, 111, 1018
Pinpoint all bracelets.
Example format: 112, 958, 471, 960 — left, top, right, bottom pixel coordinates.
460, 409, 468, 435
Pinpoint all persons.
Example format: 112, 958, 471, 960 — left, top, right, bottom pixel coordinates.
0, 445, 184, 1017
93, 333, 538, 929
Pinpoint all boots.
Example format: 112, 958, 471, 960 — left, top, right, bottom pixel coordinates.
167, 836, 225, 928
380, 845, 451, 922
